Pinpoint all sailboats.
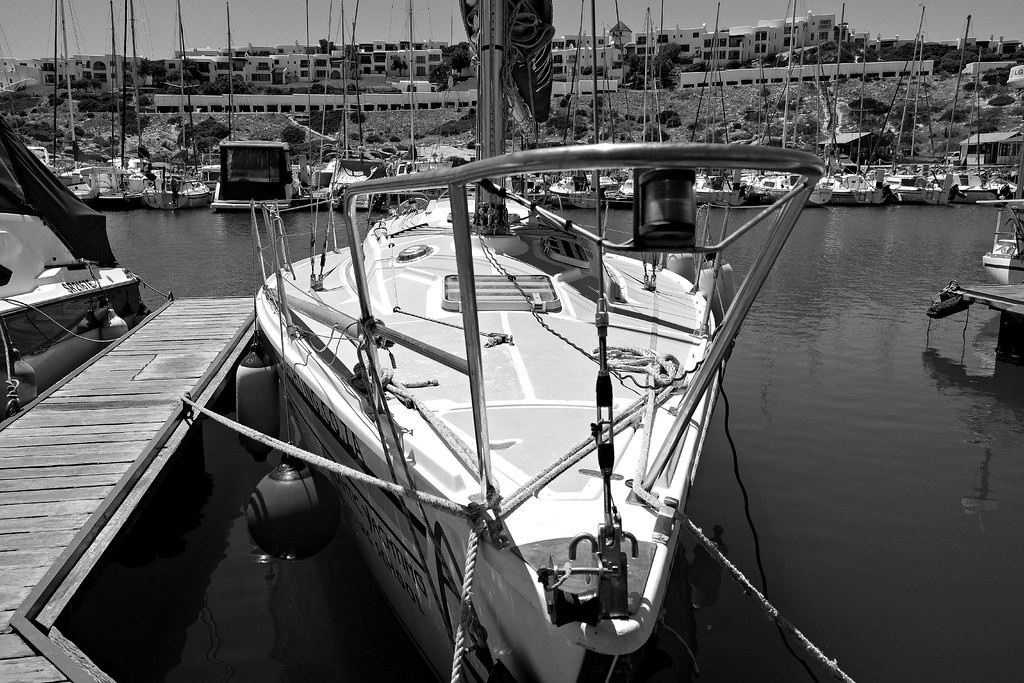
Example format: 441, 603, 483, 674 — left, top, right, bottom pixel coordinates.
6, 0, 1024, 681
